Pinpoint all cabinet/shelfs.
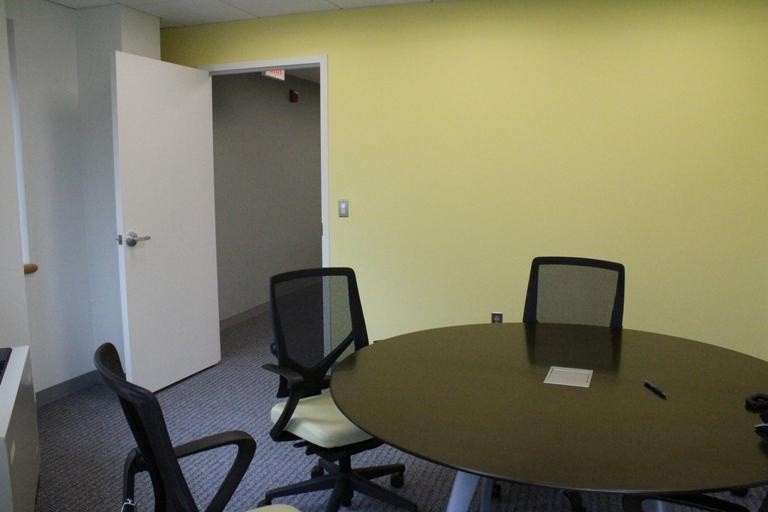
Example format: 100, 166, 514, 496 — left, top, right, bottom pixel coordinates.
0, 345, 45, 511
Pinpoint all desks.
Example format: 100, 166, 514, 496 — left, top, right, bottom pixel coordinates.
329, 322, 767, 512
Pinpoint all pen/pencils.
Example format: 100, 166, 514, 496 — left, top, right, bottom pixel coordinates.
644, 379, 668, 400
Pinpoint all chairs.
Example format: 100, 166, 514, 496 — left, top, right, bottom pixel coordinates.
619, 492, 767, 512
492, 255, 626, 512
91, 340, 308, 512
261, 263, 423, 512
